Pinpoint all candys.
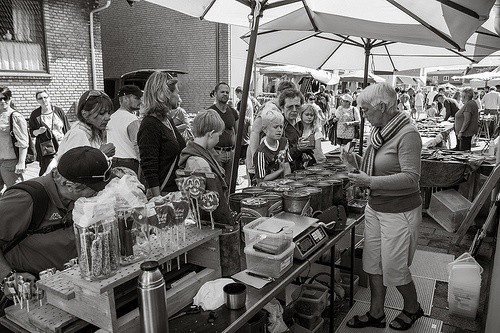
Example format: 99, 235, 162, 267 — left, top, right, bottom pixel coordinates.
129, 174, 220, 273
4, 258, 78, 313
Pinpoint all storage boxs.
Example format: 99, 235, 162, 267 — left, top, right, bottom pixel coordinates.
292, 309, 322, 331
291, 283, 329, 316
425, 188, 475, 233
241, 217, 296, 279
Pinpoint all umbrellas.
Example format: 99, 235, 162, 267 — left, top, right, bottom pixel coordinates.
241, 0, 500, 154
452, 72, 500, 90
119, 0, 497, 194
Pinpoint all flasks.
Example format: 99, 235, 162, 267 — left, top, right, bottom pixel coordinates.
137, 260, 169, 333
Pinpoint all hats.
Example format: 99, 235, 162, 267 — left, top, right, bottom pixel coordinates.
342, 94, 353, 103
117, 85, 143, 97
58, 146, 116, 192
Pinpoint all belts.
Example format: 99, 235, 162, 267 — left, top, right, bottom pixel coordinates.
215, 147, 234, 151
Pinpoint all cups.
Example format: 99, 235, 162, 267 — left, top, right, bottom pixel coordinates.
228, 157, 349, 216
223, 282, 247, 309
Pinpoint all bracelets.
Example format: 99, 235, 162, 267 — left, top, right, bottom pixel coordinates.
459, 130, 464, 133
0, 269, 17, 289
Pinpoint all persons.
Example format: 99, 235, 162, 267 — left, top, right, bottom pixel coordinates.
204, 80, 362, 188
40, 89, 116, 176
0, 146, 113, 317
179, 109, 239, 228
394, 82, 500, 152
137, 72, 195, 201
31, 90, 71, 177
105, 87, 144, 176
0, 87, 29, 196
340, 83, 424, 330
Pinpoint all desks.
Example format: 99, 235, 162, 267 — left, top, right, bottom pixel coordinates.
169, 213, 365, 333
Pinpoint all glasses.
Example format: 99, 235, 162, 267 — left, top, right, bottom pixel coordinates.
84, 90, 109, 107
37, 97, 49, 100
0, 97, 9, 101
362, 103, 381, 115
71, 152, 112, 182
166, 72, 173, 83
308, 98, 315, 101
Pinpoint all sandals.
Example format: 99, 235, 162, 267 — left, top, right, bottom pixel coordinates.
389, 301, 424, 331
347, 311, 386, 328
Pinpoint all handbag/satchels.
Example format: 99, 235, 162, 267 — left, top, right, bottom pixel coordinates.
354, 125, 359, 139
40, 139, 56, 156
9, 111, 34, 164
